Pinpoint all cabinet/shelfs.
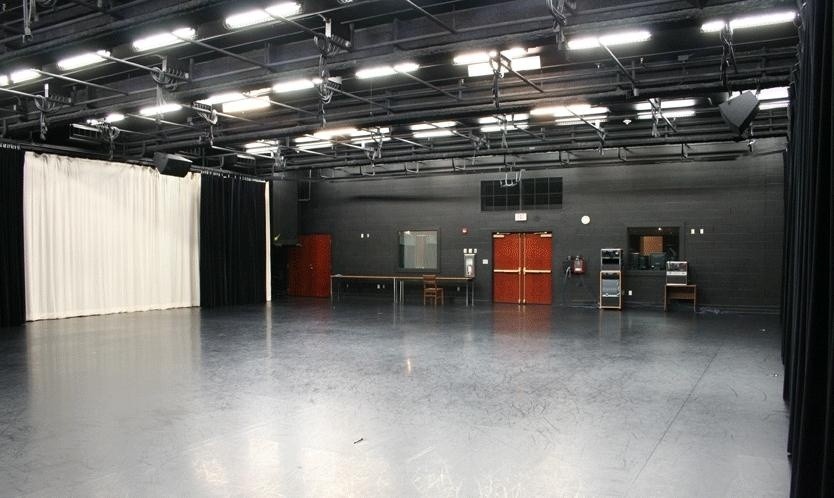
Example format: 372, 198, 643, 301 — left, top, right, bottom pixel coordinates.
599, 248, 624, 311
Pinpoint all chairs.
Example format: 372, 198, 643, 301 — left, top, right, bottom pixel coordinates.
423, 274, 444, 306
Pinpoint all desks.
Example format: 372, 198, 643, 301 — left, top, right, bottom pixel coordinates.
664, 284, 698, 314
330, 275, 475, 307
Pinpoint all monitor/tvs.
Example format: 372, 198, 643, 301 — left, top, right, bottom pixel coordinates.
649, 252, 667, 271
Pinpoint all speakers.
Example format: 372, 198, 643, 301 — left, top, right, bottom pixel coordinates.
273, 179, 301, 247
718, 91, 760, 135
153, 152, 193, 178
